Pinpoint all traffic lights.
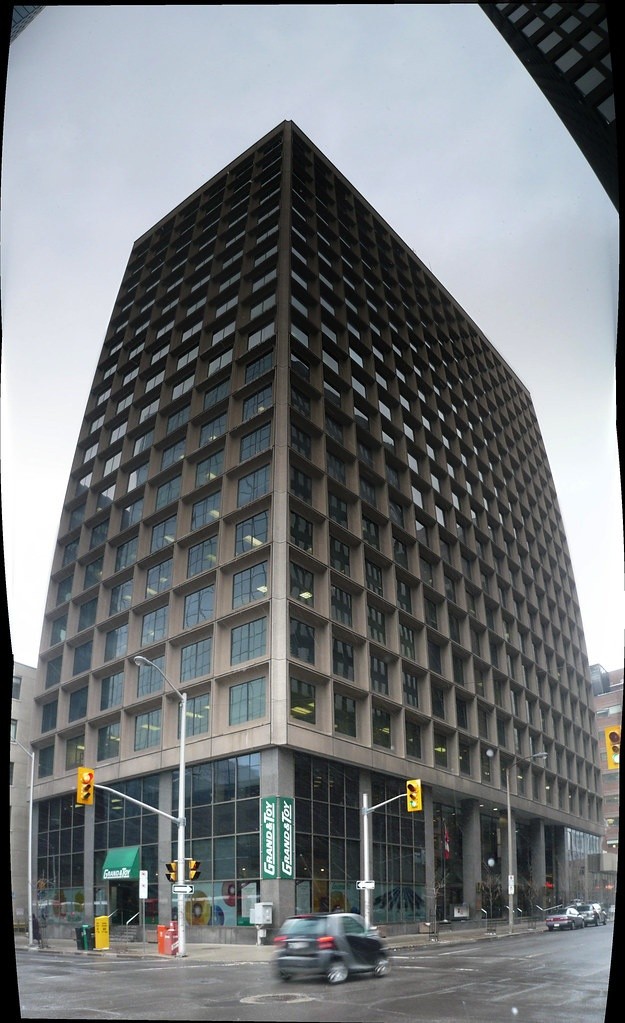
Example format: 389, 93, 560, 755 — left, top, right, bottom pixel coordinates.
165, 858, 201, 883
77, 767, 95, 805
406, 779, 422, 812
604, 725, 622, 769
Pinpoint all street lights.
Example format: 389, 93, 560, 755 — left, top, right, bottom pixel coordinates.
506, 752, 548, 933
134, 655, 187, 956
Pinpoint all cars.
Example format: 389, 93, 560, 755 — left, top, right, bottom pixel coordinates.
272, 913, 392, 985
35, 899, 108, 925
546, 907, 585, 931
576, 903, 607, 927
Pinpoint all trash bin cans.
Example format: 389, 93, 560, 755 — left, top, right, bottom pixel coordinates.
156, 920, 178, 955
75, 926, 94, 950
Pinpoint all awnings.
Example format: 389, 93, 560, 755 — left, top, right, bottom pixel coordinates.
100, 847, 139, 879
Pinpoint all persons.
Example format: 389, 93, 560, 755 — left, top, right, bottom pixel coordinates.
33, 913, 39, 939
